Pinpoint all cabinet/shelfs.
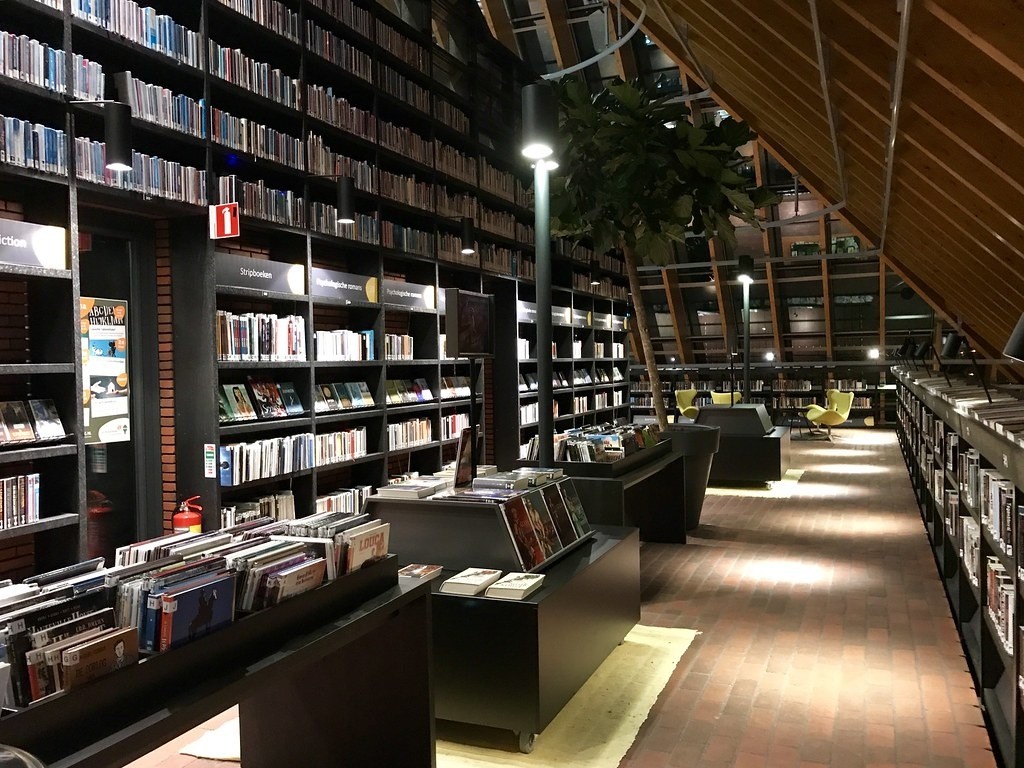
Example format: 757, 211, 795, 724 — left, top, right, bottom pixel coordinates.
2, 1, 1021, 766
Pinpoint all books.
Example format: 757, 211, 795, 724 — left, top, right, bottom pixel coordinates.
890, 384, 1024, 699
631, 380, 871, 408
0, 0, 630, 706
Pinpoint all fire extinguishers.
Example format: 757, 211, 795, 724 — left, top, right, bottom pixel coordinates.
87, 490, 113, 565
173, 495, 201, 533
